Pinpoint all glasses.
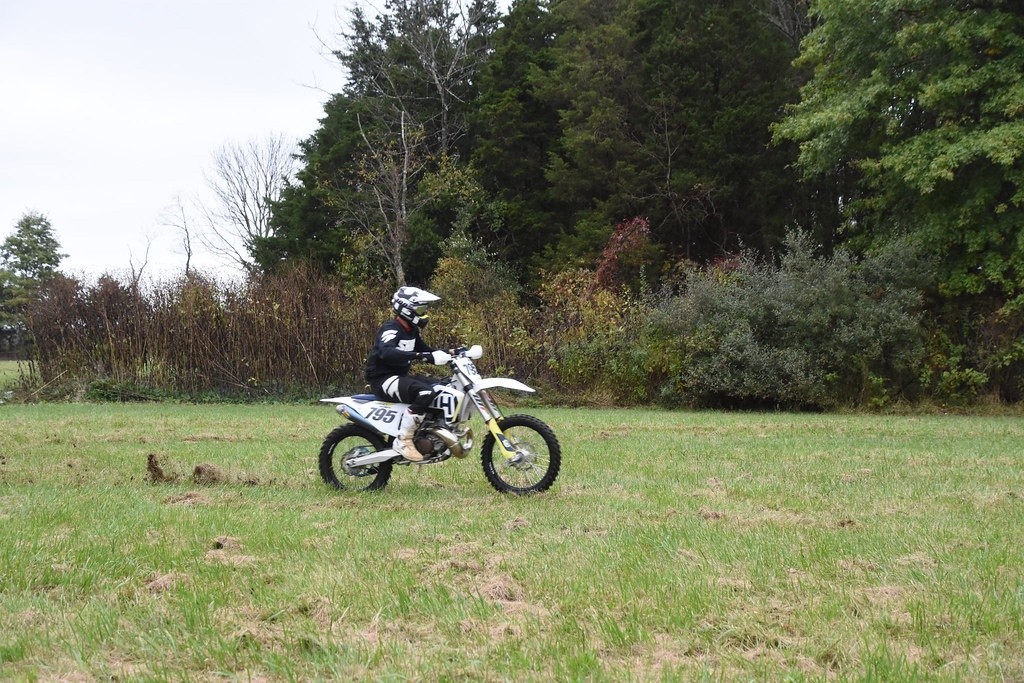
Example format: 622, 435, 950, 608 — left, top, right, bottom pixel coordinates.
411, 303, 428, 316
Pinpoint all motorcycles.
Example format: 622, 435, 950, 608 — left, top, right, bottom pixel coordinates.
317, 345, 562, 498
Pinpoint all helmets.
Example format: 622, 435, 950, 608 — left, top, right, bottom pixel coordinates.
391, 286, 441, 328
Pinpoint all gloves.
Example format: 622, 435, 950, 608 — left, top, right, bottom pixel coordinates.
417, 352, 434, 365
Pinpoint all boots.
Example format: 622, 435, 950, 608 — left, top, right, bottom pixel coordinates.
393, 410, 427, 462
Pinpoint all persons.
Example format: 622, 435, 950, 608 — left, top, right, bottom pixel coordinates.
363, 286, 466, 461
